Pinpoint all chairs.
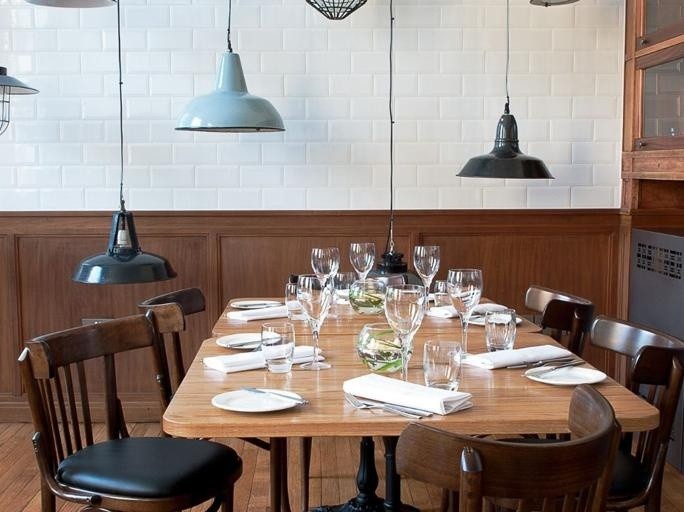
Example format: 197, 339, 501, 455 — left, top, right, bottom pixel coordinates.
395, 384, 622, 510
523, 285, 594, 363
17, 315, 243, 512
552, 314, 684, 512
139, 287, 206, 384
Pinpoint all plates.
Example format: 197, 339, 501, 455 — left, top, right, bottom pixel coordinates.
211, 389, 301, 413
216, 333, 281, 349
526, 366, 607, 386
230, 301, 280, 309
467, 314, 522, 326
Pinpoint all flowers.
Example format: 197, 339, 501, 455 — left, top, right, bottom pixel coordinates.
359, 329, 408, 372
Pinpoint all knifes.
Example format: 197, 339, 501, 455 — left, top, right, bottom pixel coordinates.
356, 399, 433, 417
240, 386, 311, 404
521, 361, 586, 377
508, 357, 573, 369
229, 337, 288, 347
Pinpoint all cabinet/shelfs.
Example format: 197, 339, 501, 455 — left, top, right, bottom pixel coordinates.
622, 0, 684, 182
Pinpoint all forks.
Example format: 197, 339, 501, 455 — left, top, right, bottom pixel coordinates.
344, 393, 423, 420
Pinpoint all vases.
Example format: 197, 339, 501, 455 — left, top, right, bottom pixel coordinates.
355, 322, 414, 373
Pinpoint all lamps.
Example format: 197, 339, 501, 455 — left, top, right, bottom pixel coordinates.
304, 0, 368, 21
0, 66, 40, 138
454, 1, 556, 180
174, 0, 286, 133
70, 1, 178, 286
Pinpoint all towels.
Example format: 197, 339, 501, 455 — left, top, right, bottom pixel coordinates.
467, 344, 571, 370
227, 306, 289, 322
203, 345, 325, 374
428, 303, 508, 319
343, 373, 473, 416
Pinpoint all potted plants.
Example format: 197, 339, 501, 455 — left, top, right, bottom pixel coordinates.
348, 279, 387, 315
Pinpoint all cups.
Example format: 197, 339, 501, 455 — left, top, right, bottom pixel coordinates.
335, 272, 356, 305
434, 280, 452, 307
447, 269, 483, 357
297, 275, 334, 370
413, 246, 440, 314
384, 284, 427, 382
311, 247, 340, 296
286, 283, 306, 320
423, 341, 461, 392
261, 323, 295, 374
485, 309, 517, 353
350, 242, 375, 280
